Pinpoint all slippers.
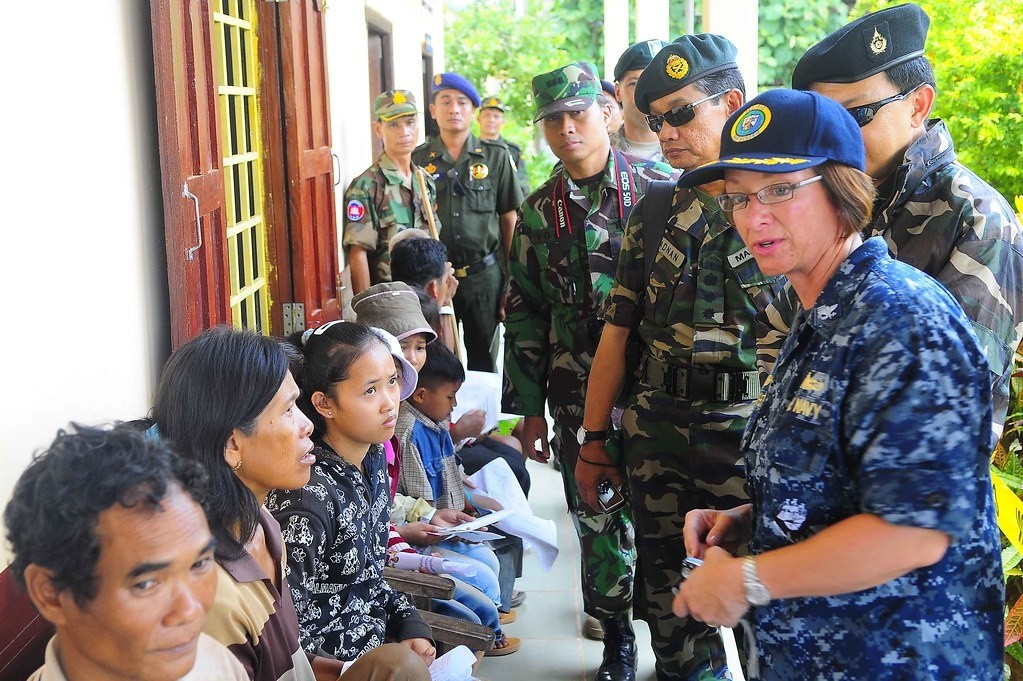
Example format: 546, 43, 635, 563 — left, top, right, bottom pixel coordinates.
509, 589, 524, 608
485, 635, 520, 658
495, 607, 513, 623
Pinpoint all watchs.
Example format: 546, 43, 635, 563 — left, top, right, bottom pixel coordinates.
741, 555, 770, 607
577, 426, 606, 445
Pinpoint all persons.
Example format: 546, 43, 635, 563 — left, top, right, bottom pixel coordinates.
756, 3, 1023, 453
263, 319, 436, 670
154, 327, 432, 681
351, 280, 501, 677
388, 227, 522, 454
583, 39, 685, 639
390, 237, 529, 607
575, 34, 788, 681
501, 62, 682, 681
600, 81, 624, 134
672, 90, 1004, 681
477, 97, 529, 198
394, 340, 519, 656
411, 73, 525, 373
343, 91, 441, 296
2, 422, 250, 681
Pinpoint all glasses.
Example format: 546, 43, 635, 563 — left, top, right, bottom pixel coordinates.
645, 84, 738, 134
716, 173, 823, 213
848, 80, 926, 129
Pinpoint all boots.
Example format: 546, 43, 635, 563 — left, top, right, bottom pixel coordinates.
597, 613, 637, 681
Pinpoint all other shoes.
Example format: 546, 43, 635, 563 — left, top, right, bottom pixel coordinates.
586, 615, 603, 640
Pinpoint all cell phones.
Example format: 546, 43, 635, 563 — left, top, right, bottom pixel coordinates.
597, 481, 626, 515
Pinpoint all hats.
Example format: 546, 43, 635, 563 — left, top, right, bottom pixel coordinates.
478, 98, 506, 113
599, 79, 621, 106
614, 42, 670, 80
369, 326, 419, 402
793, 4, 929, 91
634, 34, 737, 115
351, 286, 438, 344
678, 90, 865, 188
431, 71, 481, 108
532, 62, 603, 126
376, 89, 418, 122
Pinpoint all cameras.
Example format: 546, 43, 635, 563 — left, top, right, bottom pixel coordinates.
680, 557, 704, 583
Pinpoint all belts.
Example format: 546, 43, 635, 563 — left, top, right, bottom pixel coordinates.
454, 251, 496, 279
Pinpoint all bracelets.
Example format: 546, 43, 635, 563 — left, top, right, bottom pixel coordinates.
438, 306, 453, 315
